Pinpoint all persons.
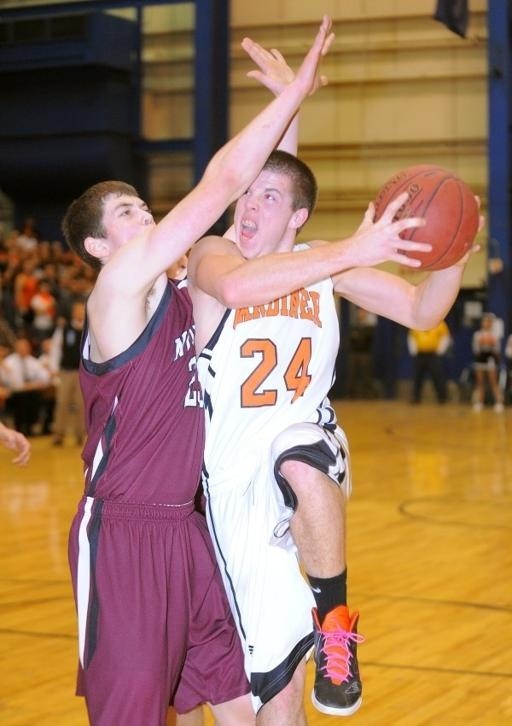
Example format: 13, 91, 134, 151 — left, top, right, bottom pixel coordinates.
60, 18, 336, 724
185, 150, 482, 724
1, 424, 32, 468
407, 302, 512, 412
3, 221, 98, 442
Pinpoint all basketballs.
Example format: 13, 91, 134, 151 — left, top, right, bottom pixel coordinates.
373, 165, 479, 270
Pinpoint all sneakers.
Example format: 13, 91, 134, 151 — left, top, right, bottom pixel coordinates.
311, 606, 361, 717
